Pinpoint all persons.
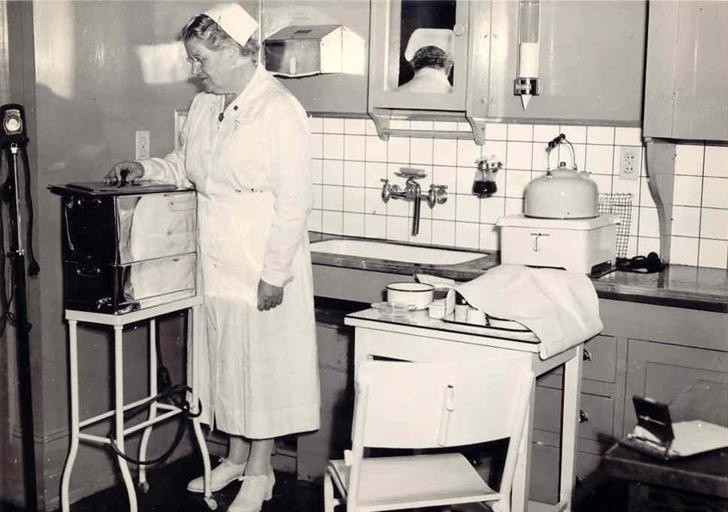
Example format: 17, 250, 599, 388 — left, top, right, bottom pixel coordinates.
102, 2, 322, 511
394, 28, 454, 92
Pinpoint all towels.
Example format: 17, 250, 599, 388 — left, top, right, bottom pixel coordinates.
415, 262, 605, 361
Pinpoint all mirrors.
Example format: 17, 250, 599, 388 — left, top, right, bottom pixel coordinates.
397, 0, 456, 99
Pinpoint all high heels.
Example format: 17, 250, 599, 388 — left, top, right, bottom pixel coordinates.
187, 456, 248, 494
226, 466, 276, 512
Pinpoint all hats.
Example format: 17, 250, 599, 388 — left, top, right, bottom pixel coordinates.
201, 1, 261, 48
404, 27, 455, 64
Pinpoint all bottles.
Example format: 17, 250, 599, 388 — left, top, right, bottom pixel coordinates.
517, 1, 539, 110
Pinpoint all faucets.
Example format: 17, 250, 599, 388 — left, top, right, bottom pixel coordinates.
405, 177, 421, 239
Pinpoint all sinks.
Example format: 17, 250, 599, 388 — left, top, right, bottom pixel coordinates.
308, 237, 490, 266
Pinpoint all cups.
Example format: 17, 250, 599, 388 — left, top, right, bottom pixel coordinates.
455, 305, 487, 325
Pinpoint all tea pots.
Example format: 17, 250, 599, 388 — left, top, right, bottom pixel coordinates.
523, 132, 600, 219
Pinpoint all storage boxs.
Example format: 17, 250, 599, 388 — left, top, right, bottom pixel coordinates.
495, 212, 620, 279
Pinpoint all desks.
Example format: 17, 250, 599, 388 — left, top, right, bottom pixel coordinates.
342, 291, 585, 512
604, 378, 726, 512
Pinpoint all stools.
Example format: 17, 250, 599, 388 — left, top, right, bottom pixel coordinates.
58, 306, 217, 512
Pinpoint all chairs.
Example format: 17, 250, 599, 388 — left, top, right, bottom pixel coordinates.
323, 354, 536, 512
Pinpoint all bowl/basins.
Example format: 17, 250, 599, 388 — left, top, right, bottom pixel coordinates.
385, 281, 434, 308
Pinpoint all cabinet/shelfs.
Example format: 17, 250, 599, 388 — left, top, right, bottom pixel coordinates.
642, 3, 728, 146
494, 0, 646, 128
526, 321, 617, 510
624, 333, 727, 498
47, 178, 198, 316
370, 1, 487, 146
298, 326, 352, 482
260, 0, 370, 117
